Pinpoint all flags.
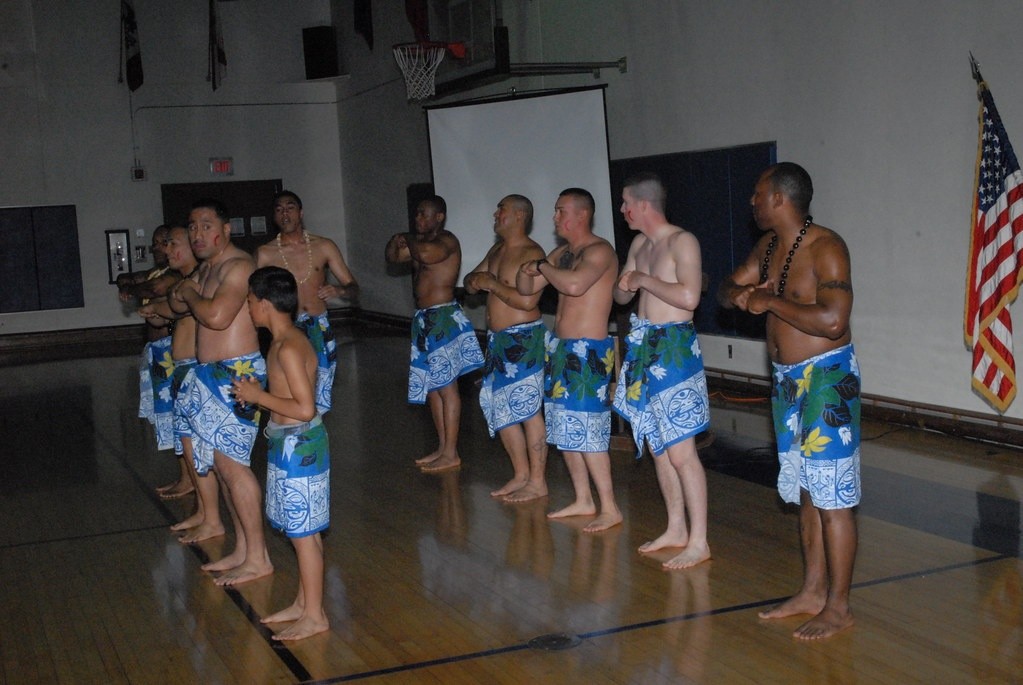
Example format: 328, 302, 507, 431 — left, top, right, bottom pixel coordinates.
963, 82, 1023, 412
124, 0, 145, 91
207, 1, 229, 91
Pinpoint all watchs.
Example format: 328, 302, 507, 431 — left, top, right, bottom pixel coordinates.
536, 259, 548, 272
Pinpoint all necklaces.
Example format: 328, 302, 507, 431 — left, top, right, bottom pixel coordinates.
760, 214, 813, 295
142, 266, 169, 305
277, 230, 313, 284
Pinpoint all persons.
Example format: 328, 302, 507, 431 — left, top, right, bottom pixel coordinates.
463, 194, 549, 504
384, 195, 486, 471
612, 173, 713, 570
515, 188, 623, 533
226, 266, 332, 643
717, 162, 863, 639
116, 189, 360, 586
